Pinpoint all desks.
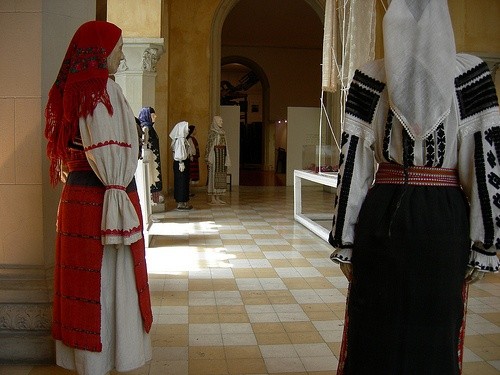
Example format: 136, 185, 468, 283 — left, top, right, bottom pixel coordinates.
293, 170, 375, 242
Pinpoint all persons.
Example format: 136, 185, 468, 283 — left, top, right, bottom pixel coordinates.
205, 116, 231, 206
169, 121, 193, 209
137, 106, 162, 204
45, 21, 157, 375
331, 0, 500, 373
185, 125, 201, 196
